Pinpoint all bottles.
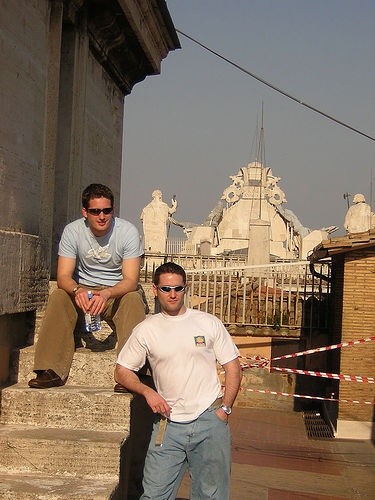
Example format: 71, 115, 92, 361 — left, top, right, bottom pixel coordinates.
84, 291, 102, 332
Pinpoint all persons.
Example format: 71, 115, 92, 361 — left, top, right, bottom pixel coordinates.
344, 193, 375, 234
28, 184, 147, 393
114, 262, 243, 500
141, 190, 177, 252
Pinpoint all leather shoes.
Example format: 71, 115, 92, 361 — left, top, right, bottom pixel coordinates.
114, 377, 146, 393
28, 369, 69, 388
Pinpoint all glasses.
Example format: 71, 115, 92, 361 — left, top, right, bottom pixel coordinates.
157, 285, 185, 293
87, 207, 113, 216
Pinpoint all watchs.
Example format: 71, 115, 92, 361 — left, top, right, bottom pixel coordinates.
72, 285, 82, 297
221, 404, 231, 415
